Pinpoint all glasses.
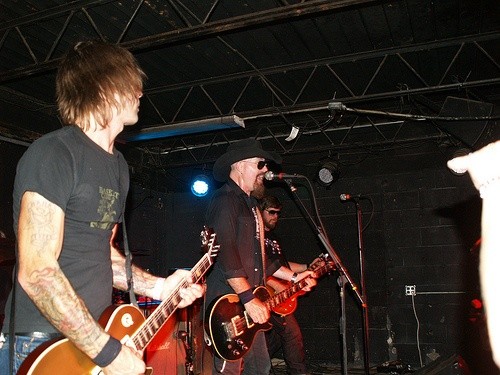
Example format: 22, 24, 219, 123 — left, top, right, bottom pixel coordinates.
242, 160, 270, 170
264, 209, 282, 215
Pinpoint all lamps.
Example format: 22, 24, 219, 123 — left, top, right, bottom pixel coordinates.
190, 165, 212, 198
315, 157, 344, 190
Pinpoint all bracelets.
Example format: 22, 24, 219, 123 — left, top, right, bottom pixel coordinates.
290, 271, 298, 283
92, 334, 122, 368
236, 289, 256, 305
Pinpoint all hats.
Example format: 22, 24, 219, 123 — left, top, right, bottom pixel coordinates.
213, 138, 284, 182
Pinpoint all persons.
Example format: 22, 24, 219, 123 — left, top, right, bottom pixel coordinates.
203, 140, 317, 375
0, 39, 206, 375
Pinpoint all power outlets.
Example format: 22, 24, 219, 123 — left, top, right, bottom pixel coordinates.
405, 285, 416, 296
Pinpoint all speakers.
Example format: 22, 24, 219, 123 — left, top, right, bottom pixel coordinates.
412, 351, 470, 375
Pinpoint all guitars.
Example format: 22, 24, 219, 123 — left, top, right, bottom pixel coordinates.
265, 253, 328, 316
16, 223, 221, 375
204, 253, 336, 362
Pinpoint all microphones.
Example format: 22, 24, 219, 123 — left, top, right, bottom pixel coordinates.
264, 170, 307, 181
340, 193, 368, 200
